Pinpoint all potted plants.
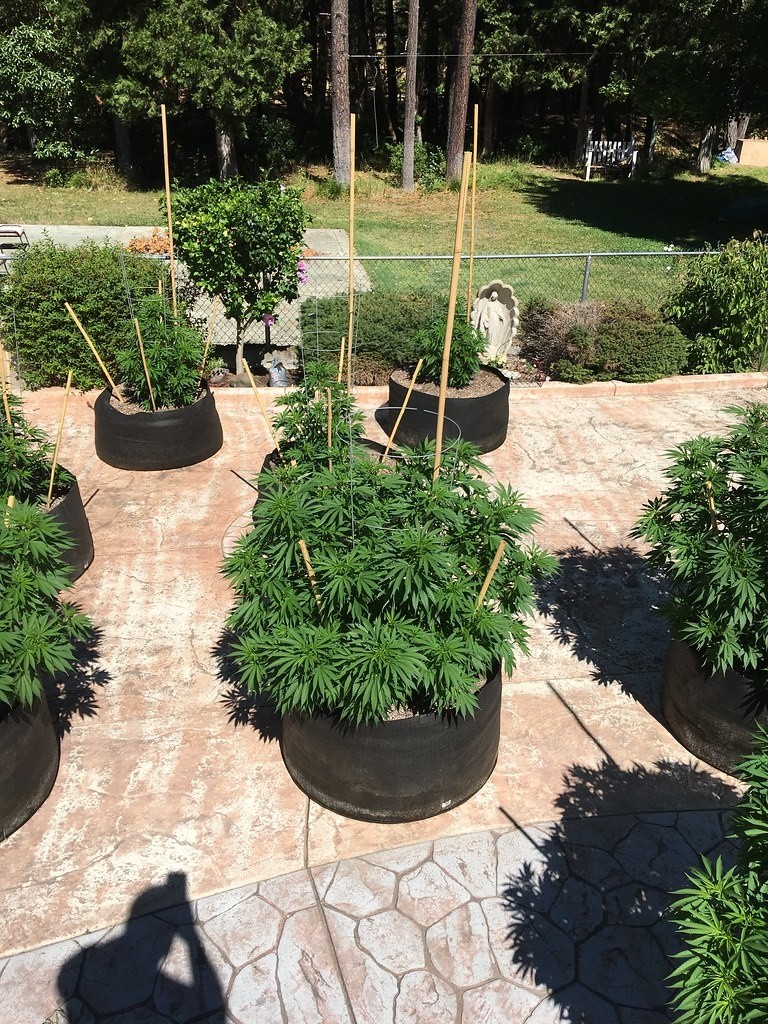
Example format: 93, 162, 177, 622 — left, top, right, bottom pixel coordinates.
0, 502, 103, 839
619, 401, 768, 784
386, 298, 512, 455
94, 295, 224, 471
227, 361, 567, 818
0, 391, 94, 587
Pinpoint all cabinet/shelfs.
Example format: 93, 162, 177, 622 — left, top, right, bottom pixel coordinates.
0, 224, 28, 275
